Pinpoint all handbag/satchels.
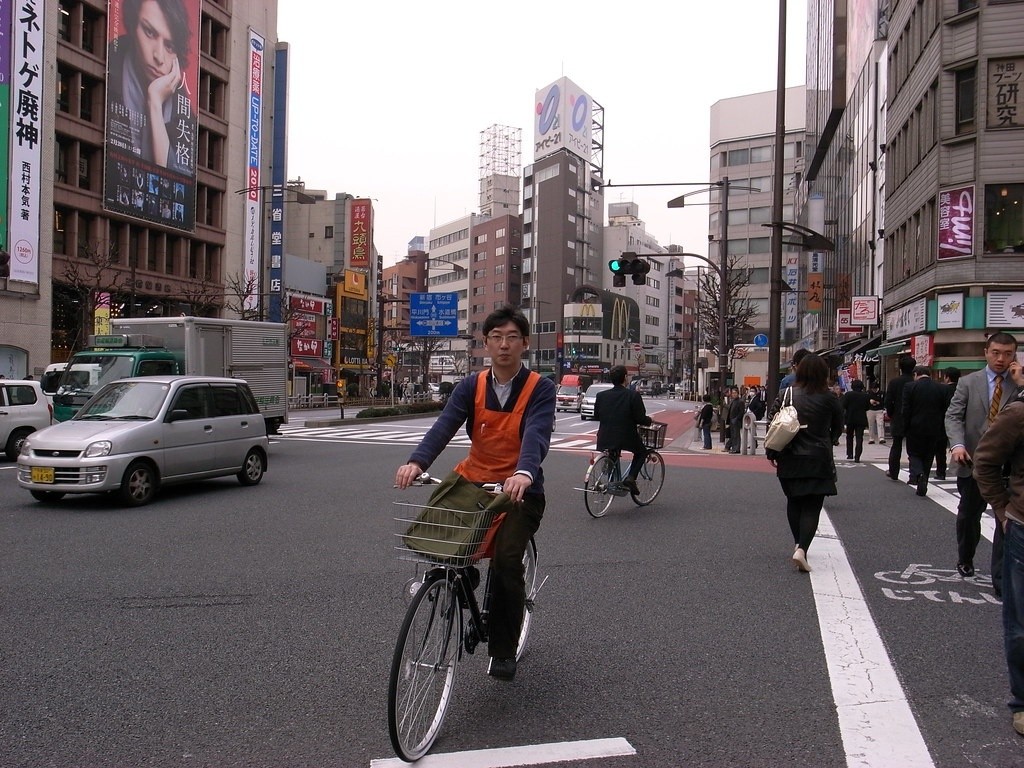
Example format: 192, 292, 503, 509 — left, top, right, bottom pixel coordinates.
403, 471, 515, 563
764, 387, 808, 451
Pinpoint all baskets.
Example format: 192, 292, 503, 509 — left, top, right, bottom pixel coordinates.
393, 495, 498, 568
638, 421, 668, 449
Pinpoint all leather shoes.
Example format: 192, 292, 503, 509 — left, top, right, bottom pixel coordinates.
907, 480, 916, 485
934, 476, 945, 480
623, 476, 640, 495
886, 470, 898, 480
957, 559, 974, 576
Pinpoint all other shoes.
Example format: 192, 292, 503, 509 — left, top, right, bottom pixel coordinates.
794, 544, 799, 552
868, 441, 875, 444
793, 548, 812, 572
490, 657, 518, 680
728, 451, 739, 454
1013, 712, 1024, 734
721, 448, 730, 452
847, 455, 853, 459
458, 566, 479, 598
879, 440, 886, 444
854, 458, 860, 462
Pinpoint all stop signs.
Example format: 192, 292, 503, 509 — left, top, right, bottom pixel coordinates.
634, 345, 640, 351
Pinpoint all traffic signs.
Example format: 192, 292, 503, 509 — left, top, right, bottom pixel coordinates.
409, 292, 459, 338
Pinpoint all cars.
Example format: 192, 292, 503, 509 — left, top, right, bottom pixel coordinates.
427, 382, 440, 393
633, 378, 695, 396
0, 362, 271, 506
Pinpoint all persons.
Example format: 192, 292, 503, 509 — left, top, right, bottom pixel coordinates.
973, 397, 1024, 734
765, 349, 844, 572
395, 306, 556, 681
885, 356, 961, 496
389, 379, 403, 401
109, 0, 196, 176
944, 333, 1024, 597
594, 365, 655, 495
866, 383, 886, 444
651, 385, 658, 398
832, 380, 871, 463
698, 384, 768, 454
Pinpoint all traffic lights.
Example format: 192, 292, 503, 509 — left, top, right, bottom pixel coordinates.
630, 274, 646, 285
337, 380, 346, 398
608, 259, 650, 274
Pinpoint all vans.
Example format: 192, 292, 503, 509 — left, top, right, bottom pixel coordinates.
580, 384, 615, 421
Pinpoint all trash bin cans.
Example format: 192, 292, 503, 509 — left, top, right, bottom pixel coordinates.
323, 381, 338, 401
415, 384, 423, 399
439, 382, 453, 399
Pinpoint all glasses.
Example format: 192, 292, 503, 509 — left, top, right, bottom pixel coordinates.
487, 335, 524, 343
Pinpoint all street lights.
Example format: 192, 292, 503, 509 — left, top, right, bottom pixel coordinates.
421, 258, 464, 395
665, 265, 706, 402
666, 184, 729, 402
762, 220, 834, 433
259, 185, 326, 317
518, 303, 540, 374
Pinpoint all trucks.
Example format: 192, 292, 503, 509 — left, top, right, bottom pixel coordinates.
555, 375, 592, 413
48, 317, 289, 433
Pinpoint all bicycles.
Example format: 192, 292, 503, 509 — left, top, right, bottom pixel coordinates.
573, 417, 669, 519
386, 471, 550, 764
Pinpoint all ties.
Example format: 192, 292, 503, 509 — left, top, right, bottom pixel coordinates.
989, 376, 1002, 425
